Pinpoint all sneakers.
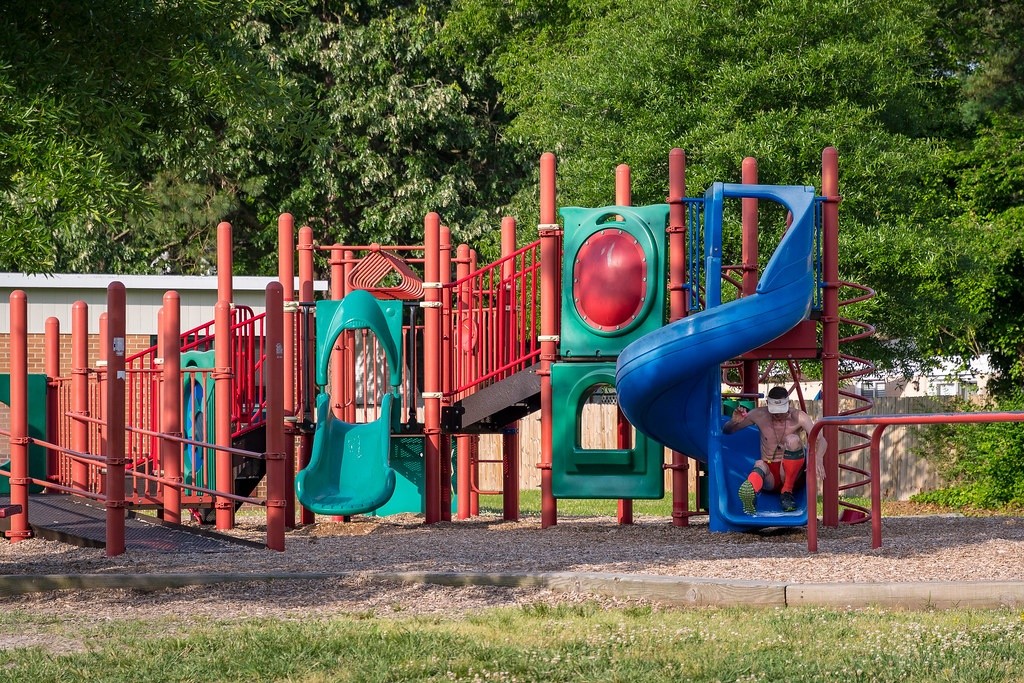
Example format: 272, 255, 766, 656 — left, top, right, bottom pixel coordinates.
780, 491, 798, 511
739, 480, 758, 517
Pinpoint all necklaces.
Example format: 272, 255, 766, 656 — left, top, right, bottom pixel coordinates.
772, 416, 786, 450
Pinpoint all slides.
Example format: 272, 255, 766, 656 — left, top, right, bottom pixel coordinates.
611, 273, 818, 533
293, 394, 396, 515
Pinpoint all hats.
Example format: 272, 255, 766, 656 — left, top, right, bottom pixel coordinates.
765, 396, 790, 414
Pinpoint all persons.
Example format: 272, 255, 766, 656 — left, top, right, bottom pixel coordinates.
722, 387, 827, 516
